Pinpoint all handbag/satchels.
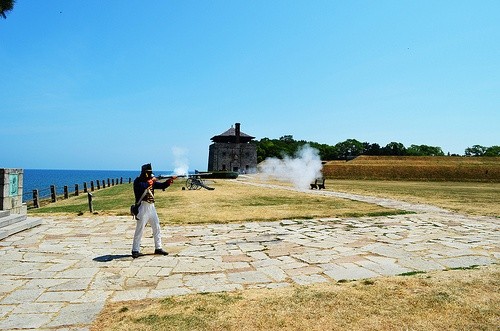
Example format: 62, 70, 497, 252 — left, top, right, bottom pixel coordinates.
131, 204, 140, 221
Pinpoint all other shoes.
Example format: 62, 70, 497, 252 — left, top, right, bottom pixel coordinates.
132, 251, 139, 258
155, 249, 168, 255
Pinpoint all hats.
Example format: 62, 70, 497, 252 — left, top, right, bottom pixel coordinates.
142, 164, 153, 173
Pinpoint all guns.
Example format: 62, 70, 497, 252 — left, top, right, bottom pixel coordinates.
146, 172, 213, 180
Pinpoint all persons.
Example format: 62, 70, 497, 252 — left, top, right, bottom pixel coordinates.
132, 162, 178, 259
